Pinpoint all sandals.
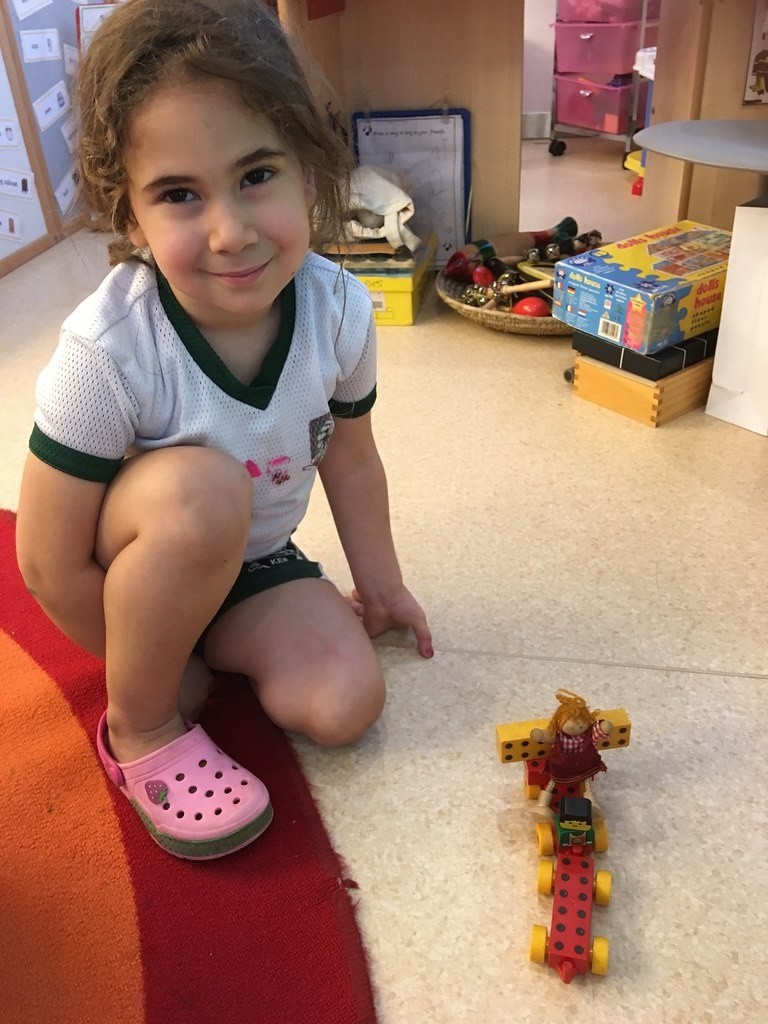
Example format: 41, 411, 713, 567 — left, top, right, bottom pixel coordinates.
95, 709, 275, 862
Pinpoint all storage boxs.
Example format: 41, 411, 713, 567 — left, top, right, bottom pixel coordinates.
705, 194, 768, 438
571, 327, 719, 382
352, 231, 439, 327
571, 357, 714, 429
554, 73, 648, 135
550, 18, 658, 74
551, 219, 732, 355
556, 0, 661, 23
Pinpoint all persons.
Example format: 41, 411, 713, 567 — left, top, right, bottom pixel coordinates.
16, 0, 434, 862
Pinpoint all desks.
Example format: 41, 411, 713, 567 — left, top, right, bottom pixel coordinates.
632, 117, 768, 175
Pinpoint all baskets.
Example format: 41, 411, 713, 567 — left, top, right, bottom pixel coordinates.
435, 259, 574, 337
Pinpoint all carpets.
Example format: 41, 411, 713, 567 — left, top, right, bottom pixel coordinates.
0, 508, 378, 1024
